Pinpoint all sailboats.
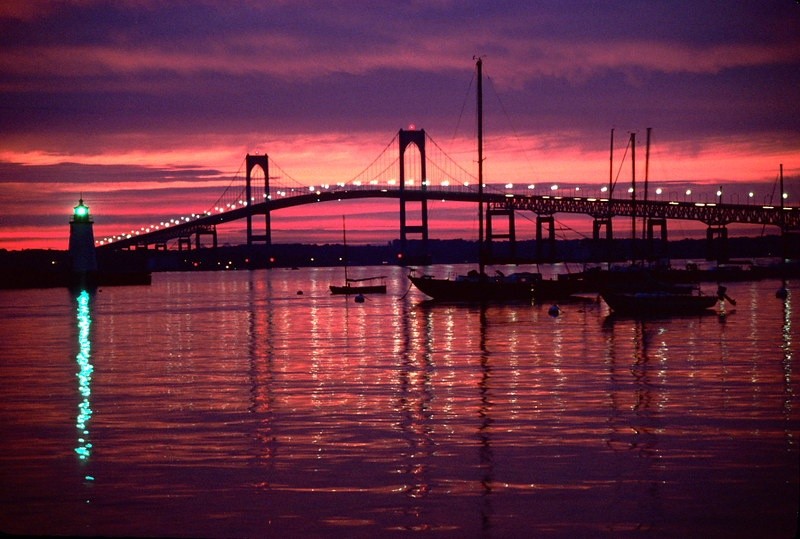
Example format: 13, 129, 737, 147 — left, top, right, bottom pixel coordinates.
574, 123, 800, 319
405, 52, 584, 308
328, 214, 388, 296
